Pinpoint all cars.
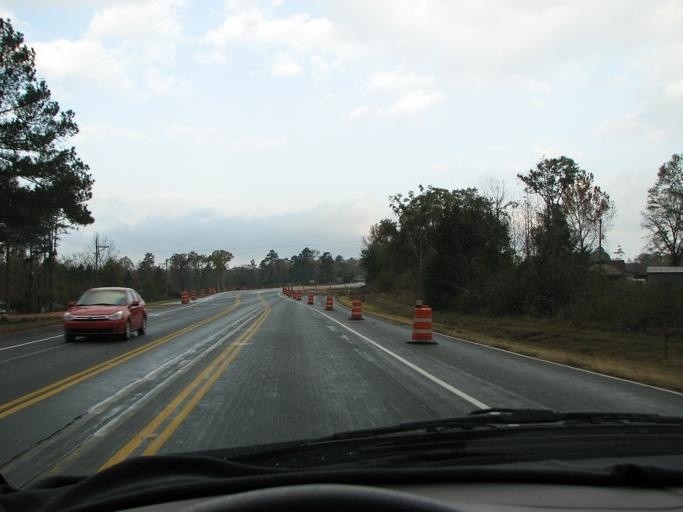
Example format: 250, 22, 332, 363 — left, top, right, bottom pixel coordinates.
61, 284, 148, 341
